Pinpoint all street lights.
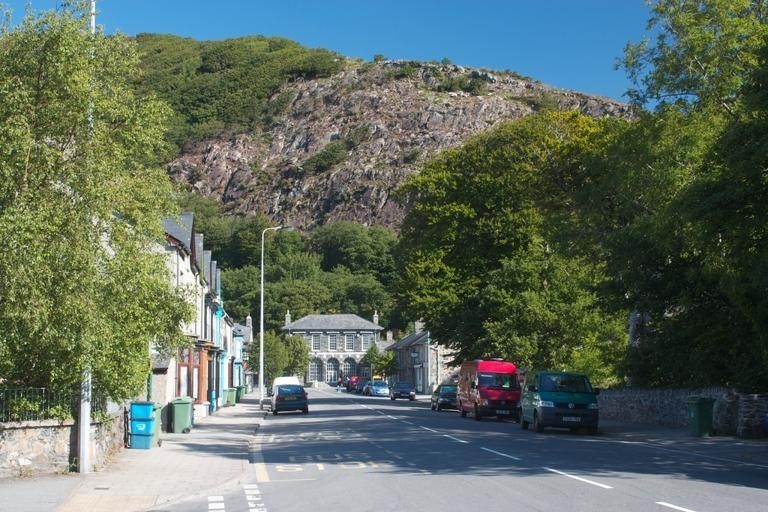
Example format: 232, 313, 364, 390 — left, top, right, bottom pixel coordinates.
258, 225, 283, 405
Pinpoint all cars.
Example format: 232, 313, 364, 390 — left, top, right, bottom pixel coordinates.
431, 384, 457, 412
270, 384, 309, 414
363, 380, 390, 397
388, 382, 416, 401
344, 376, 369, 393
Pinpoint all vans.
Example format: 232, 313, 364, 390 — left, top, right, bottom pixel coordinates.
456, 357, 521, 423
272, 376, 301, 410
519, 370, 599, 435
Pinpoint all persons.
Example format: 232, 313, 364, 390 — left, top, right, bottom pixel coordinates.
338, 375, 350, 386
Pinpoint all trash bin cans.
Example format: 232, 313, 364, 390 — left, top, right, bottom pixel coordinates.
685, 396, 716, 437
170, 396, 195, 433
128, 402, 163, 449
227, 384, 248, 406
